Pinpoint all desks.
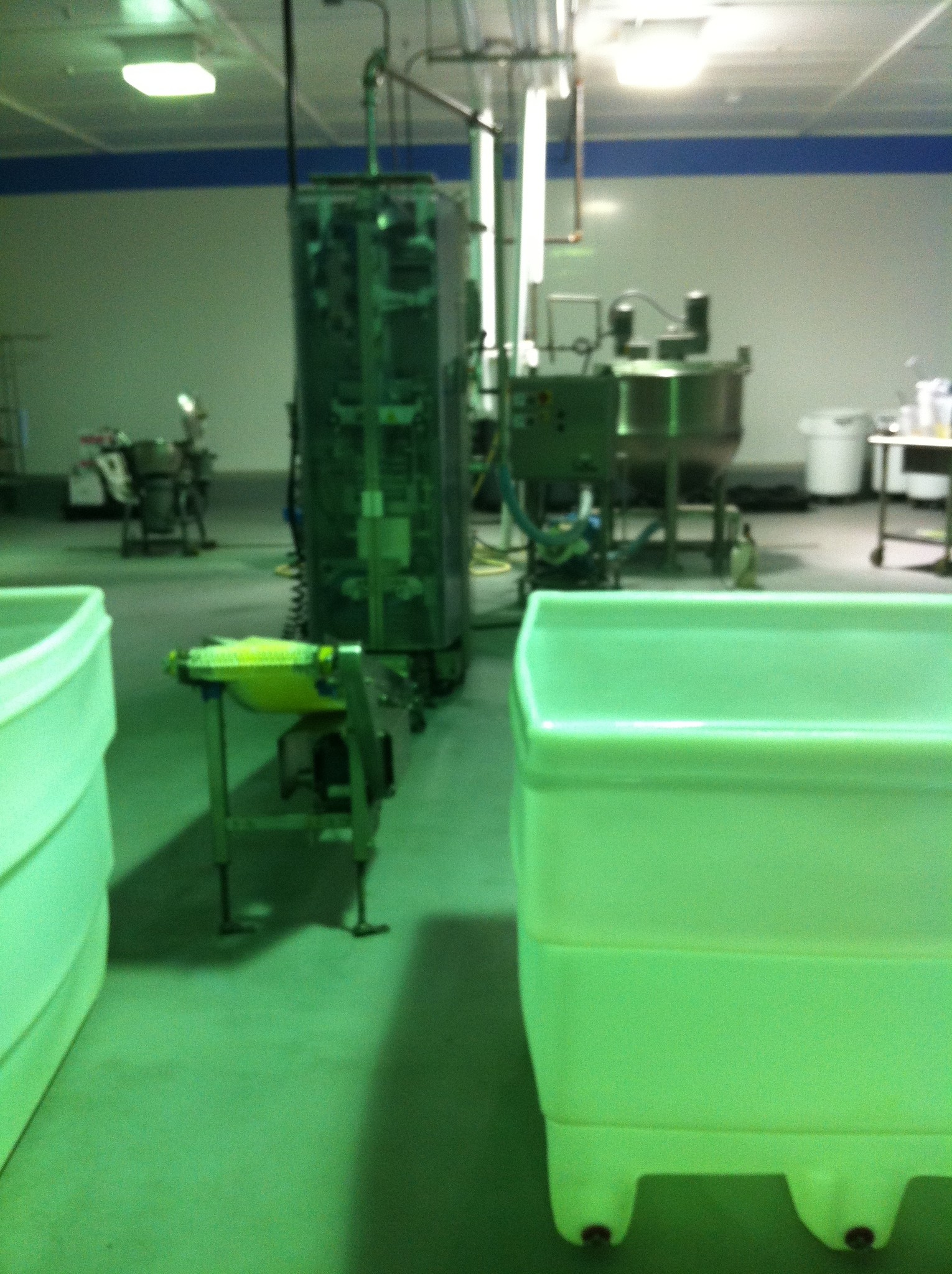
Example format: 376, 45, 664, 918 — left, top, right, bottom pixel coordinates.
868, 434, 952, 573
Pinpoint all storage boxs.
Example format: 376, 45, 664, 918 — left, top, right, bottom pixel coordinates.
0, 587, 117, 1171
506, 592, 952, 1255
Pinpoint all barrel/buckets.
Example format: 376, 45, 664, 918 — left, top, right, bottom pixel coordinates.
799, 406, 872, 498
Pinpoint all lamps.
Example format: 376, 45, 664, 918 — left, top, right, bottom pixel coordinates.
119, 37, 216, 95
